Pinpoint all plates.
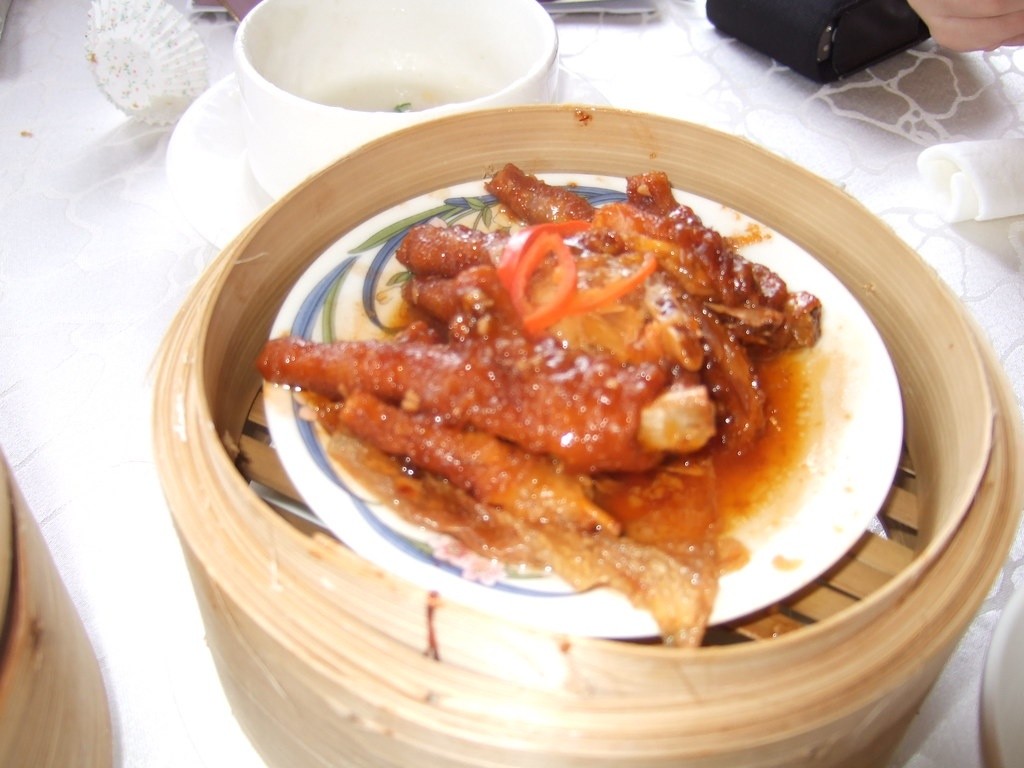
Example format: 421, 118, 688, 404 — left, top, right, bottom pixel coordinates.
977, 586, 1024, 768
261, 171, 905, 636
166, 75, 276, 250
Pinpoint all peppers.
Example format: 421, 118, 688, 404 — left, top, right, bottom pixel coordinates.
497, 218, 659, 334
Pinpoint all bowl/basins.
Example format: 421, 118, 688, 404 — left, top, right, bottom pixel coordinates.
231, 0, 560, 201
